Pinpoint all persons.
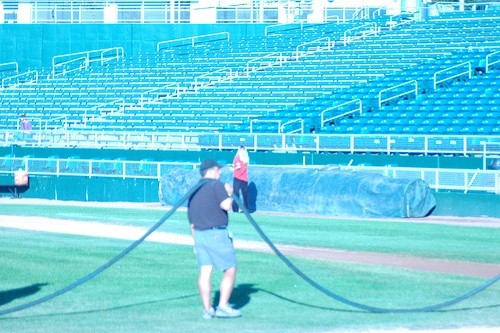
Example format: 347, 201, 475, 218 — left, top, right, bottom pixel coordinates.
187, 159, 241, 319
20, 115, 32, 130
229, 145, 249, 213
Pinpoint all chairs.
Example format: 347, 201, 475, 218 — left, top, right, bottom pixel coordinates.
0, 8, 498, 175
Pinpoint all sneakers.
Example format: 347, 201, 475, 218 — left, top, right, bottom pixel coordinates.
214, 306, 242, 317
203, 308, 215, 318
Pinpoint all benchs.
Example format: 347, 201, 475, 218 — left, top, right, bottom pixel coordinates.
0, 184, 28, 198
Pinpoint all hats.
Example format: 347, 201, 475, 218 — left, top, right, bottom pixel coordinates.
199, 159, 223, 169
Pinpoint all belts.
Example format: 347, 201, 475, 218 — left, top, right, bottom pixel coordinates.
193, 225, 226, 231
